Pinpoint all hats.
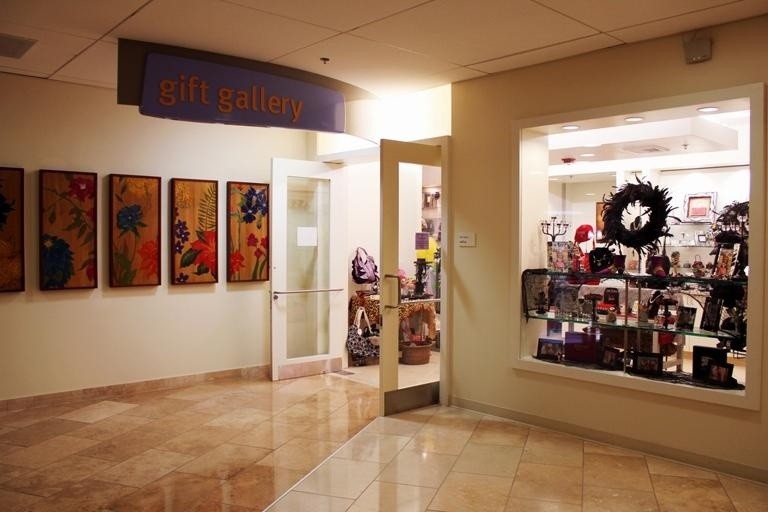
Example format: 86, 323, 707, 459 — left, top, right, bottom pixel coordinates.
575, 224, 594, 246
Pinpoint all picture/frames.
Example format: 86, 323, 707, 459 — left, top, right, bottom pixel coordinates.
1, 166, 270, 294
709, 242, 741, 280
674, 306, 697, 331
594, 201, 616, 243
700, 296, 723, 333
536, 338, 563, 361
632, 352, 664, 379
692, 345, 734, 387
597, 346, 620, 372
681, 192, 718, 223
694, 229, 713, 247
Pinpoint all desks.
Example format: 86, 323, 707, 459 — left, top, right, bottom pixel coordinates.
348, 295, 436, 352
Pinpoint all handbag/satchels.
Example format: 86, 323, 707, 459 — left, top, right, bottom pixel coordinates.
584, 248, 625, 275
352, 255, 376, 283
347, 327, 381, 357
645, 253, 670, 276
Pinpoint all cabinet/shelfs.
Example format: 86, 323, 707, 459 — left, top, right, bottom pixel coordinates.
521, 269, 746, 373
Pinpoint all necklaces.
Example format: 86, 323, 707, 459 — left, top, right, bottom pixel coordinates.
649, 292, 663, 304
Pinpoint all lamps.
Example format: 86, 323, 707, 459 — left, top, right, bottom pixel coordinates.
408, 237, 436, 299
